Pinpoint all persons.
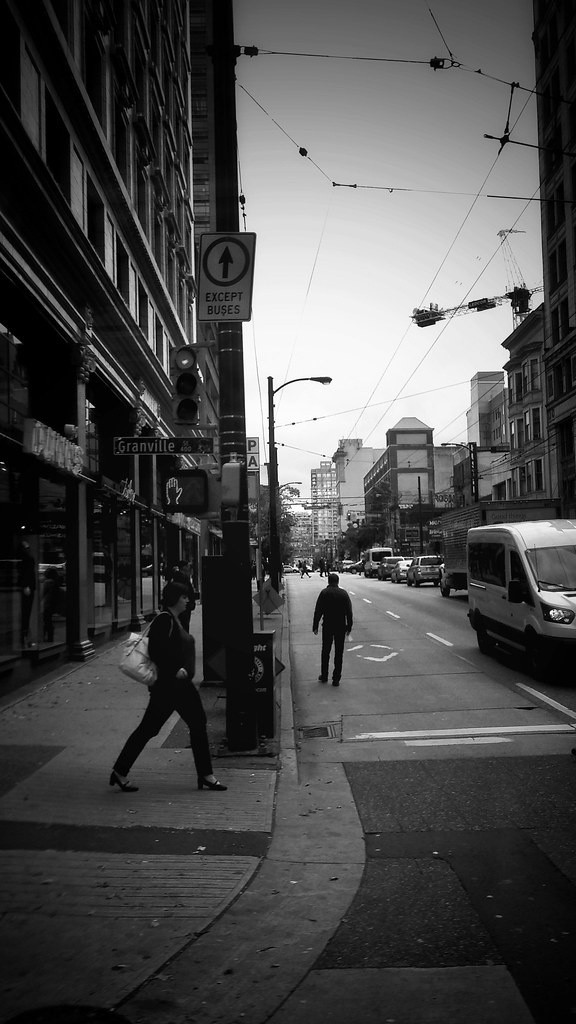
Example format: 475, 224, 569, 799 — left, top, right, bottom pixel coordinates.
172, 565, 179, 580
110, 585, 228, 793
245, 554, 285, 582
358, 555, 366, 576
325, 561, 331, 576
42, 568, 59, 639
337, 560, 344, 574
301, 561, 312, 579
319, 559, 327, 577
298, 560, 302, 576
169, 559, 199, 634
312, 573, 353, 686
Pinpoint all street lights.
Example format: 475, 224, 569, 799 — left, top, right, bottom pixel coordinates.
267, 374, 332, 594
442, 441, 479, 504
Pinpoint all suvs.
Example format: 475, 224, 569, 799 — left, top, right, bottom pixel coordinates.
377, 557, 406, 581
406, 555, 442, 588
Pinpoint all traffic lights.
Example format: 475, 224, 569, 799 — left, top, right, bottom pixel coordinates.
169, 342, 206, 427
160, 470, 207, 515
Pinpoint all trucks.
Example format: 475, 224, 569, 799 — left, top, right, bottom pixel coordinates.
439, 496, 564, 598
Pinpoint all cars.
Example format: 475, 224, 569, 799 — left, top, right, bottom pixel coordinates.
346, 560, 365, 575
391, 559, 414, 582
282, 564, 299, 572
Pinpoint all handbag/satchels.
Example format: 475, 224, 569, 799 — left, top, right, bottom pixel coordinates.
117, 611, 174, 686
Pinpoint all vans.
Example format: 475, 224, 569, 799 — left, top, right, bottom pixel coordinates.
337, 560, 354, 573
364, 547, 393, 578
467, 517, 576, 683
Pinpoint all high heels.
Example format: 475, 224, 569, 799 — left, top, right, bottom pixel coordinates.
109, 771, 138, 792
197, 776, 228, 792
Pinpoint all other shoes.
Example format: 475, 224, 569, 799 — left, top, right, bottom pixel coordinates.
317, 675, 340, 686
